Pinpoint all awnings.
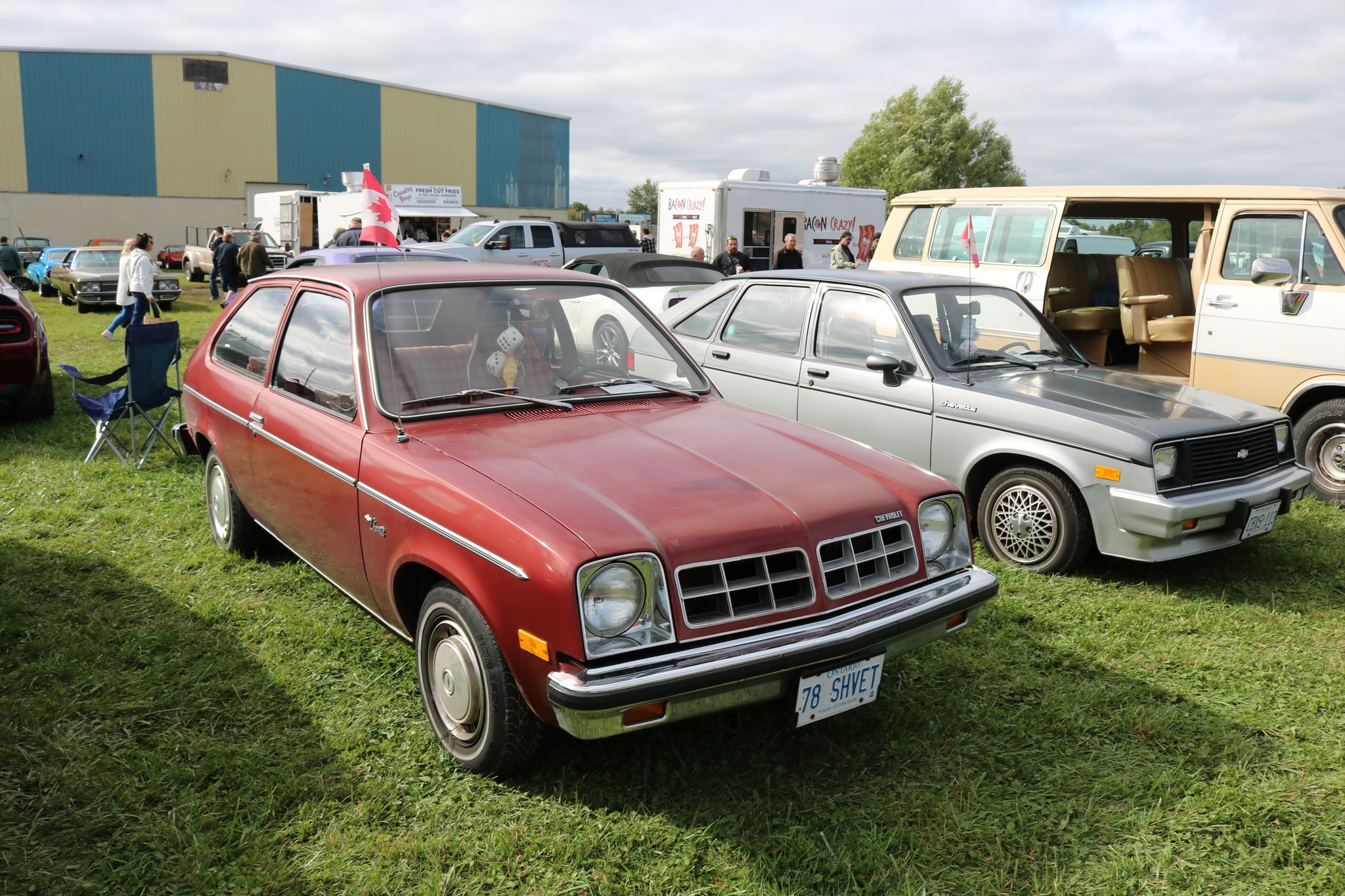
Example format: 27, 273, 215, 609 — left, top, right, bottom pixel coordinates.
394, 206, 479, 217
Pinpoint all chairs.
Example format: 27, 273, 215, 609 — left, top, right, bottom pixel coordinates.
60, 320, 182, 470
897, 314, 949, 368
467, 324, 559, 396
318, 325, 420, 415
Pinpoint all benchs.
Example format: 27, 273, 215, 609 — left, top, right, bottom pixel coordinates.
395, 341, 474, 399
1040, 248, 1195, 379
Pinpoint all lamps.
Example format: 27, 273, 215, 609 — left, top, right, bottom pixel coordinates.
324, 174, 332, 184
225, 169, 232, 180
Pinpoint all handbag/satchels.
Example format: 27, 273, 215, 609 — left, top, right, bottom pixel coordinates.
143, 300, 172, 325
127, 284, 132, 296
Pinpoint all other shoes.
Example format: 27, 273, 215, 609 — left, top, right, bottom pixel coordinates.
220, 300, 228, 309
101, 329, 115, 341
209, 296, 218, 300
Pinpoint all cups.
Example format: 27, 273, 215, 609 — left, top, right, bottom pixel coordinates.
736, 266, 743, 274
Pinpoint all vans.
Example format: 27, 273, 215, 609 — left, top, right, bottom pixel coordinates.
862, 184, 1345, 505
1029, 224, 1136, 257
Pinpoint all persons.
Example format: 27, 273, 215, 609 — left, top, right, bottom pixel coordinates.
624, 219, 651, 225
101, 233, 157, 339
773, 233, 803, 270
712, 236, 751, 276
868, 232, 882, 267
830, 231, 863, 269
585, 215, 595, 222
632, 228, 657, 253
208, 226, 274, 309
0, 236, 22, 279
398, 220, 458, 245
324, 217, 377, 249
691, 246, 706, 261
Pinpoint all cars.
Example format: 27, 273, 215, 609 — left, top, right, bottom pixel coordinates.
51, 246, 181, 314
626, 270, 1315, 575
1130, 240, 1197, 258
0, 266, 55, 424
157, 244, 186, 270
1229, 242, 1325, 274
171, 261, 1000, 785
26, 246, 109, 297
513, 252, 728, 373
83, 239, 126, 262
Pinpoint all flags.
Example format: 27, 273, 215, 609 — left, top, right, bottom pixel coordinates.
961, 216, 980, 270
359, 168, 401, 251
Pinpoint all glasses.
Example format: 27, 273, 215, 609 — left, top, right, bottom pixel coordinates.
727, 236, 737, 241
148, 241, 153, 244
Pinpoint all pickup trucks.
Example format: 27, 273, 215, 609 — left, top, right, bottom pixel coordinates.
182, 227, 295, 289
396, 220, 643, 276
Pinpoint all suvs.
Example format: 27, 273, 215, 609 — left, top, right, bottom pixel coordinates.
11, 237, 55, 272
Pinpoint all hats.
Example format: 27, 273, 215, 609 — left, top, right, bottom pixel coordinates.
640, 231, 645, 233
842, 231, 854, 239
632, 230, 636, 234
249, 231, 261, 238
351, 217, 362, 223
